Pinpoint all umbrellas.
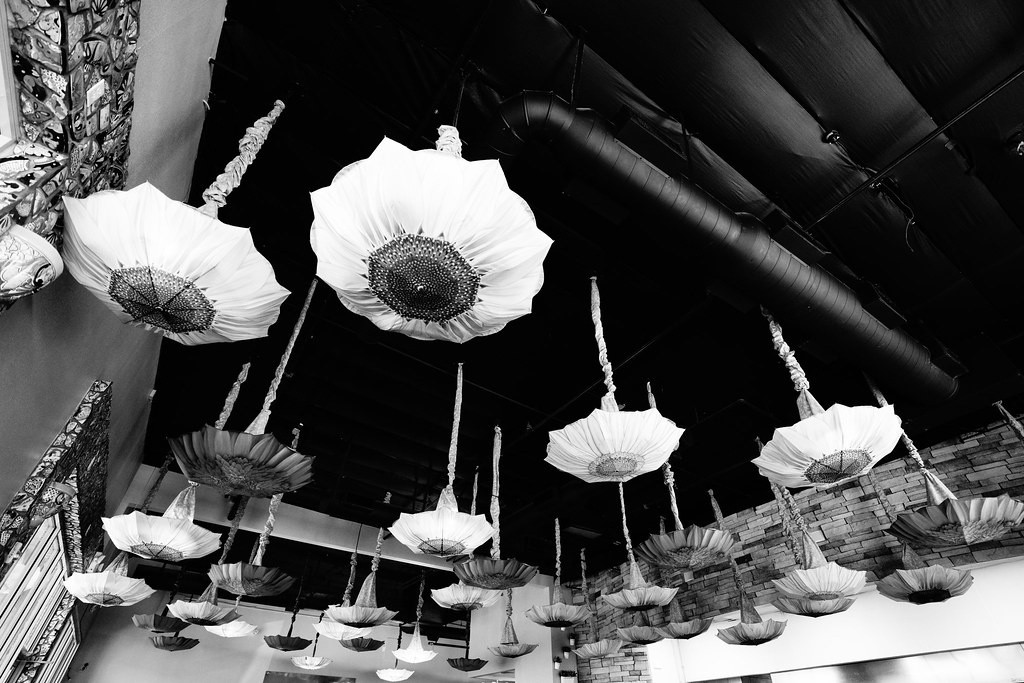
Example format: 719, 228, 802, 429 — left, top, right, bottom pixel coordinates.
309, 135, 554, 345
100, 510, 223, 561
874, 564, 974, 605
207, 561, 296, 597
62, 180, 291, 346
770, 561, 868, 618
882, 492, 1024, 547
715, 618, 788, 646
387, 511, 497, 558
751, 403, 904, 490
132, 600, 489, 683
544, 409, 685, 484
429, 524, 735, 659
63, 571, 157, 607
166, 423, 316, 498
